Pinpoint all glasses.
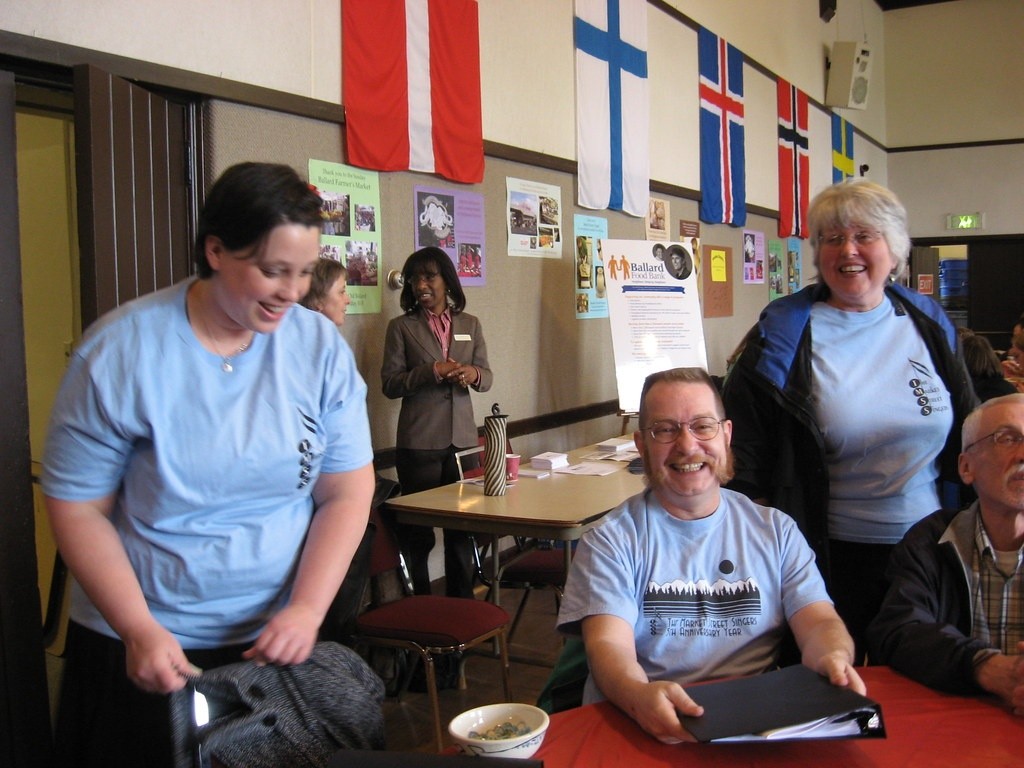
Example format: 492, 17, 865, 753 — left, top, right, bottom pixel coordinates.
964, 430, 1024, 453
407, 272, 440, 285
817, 229, 882, 246
639, 416, 726, 443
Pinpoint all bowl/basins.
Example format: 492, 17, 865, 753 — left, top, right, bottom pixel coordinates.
448, 702, 551, 759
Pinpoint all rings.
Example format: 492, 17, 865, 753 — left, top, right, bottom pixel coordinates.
461, 374, 464, 378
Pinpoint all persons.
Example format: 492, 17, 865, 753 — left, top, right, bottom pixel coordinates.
670, 247, 691, 280
555, 367, 867, 744
717, 178, 981, 669
460, 246, 482, 273
299, 259, 349, 327
379, 247, 493, 600
874, 394, 1024, 713
37, 162, 373, 768
956, 320, 1024, 403
655, 244, 665, 261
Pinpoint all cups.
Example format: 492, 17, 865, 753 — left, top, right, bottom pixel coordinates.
504, 453, 521, 482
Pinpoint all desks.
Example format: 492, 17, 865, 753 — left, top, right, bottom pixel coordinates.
382, 429, 646, 666
454, 665, 1024, 768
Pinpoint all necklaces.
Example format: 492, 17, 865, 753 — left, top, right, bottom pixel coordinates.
198, 299, 247, 373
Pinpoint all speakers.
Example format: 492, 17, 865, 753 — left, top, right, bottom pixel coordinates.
826, 43, 873, 111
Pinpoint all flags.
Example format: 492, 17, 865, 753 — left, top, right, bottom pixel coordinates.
777, 77, 811, 239
698, 26, 747, 227
574, 0, 650, 217
339, 0, 485, 182
830, 113, 855, 184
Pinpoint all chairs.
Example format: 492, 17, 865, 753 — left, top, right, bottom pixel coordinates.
452, 446, 576, 650
168, 644, 387, 768
324, 469, 513, 752
